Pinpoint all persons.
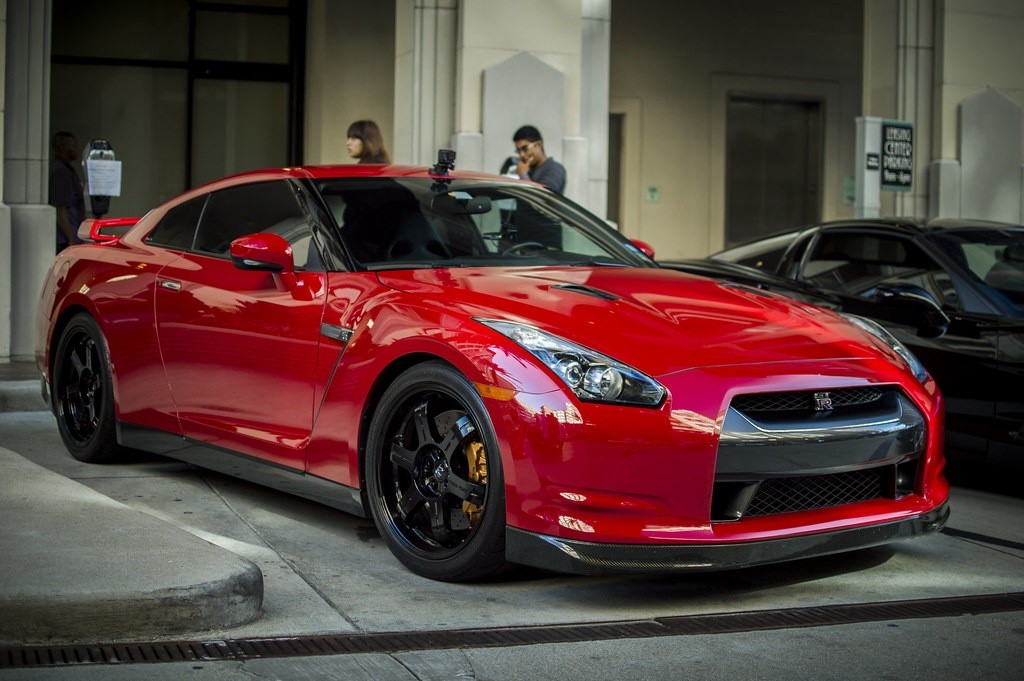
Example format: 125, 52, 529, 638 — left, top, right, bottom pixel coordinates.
513, 125, 567, 250
347, 117, 391, 165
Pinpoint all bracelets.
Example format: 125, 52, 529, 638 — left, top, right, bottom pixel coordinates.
520, 174, 529, 179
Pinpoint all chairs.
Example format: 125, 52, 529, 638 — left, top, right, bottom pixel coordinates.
358, 186, 475, 263
906, 237, 972, 274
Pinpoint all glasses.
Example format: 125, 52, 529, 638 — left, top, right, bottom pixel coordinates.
514, 141, 538, 154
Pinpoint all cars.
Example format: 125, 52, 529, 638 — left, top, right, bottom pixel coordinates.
657, 217, 1023, 484
34, 147, 953, 584
